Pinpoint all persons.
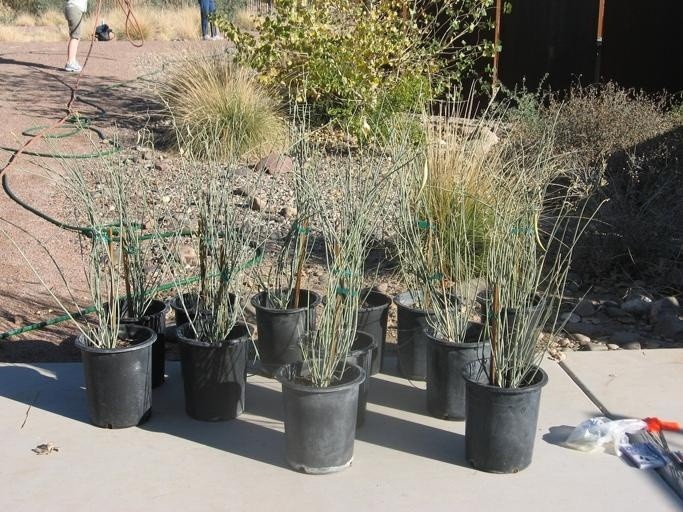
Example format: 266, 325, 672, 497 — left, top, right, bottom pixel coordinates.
65, 0, 87, 71
199, 0, 224, 40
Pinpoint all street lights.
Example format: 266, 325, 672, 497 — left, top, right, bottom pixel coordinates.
95, 25, 115, 41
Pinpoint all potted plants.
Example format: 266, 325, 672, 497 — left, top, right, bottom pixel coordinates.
0, 72, 612, 476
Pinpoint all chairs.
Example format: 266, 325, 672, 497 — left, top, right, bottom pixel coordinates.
65, 61, 82, 71
204, 35, 222, 39
